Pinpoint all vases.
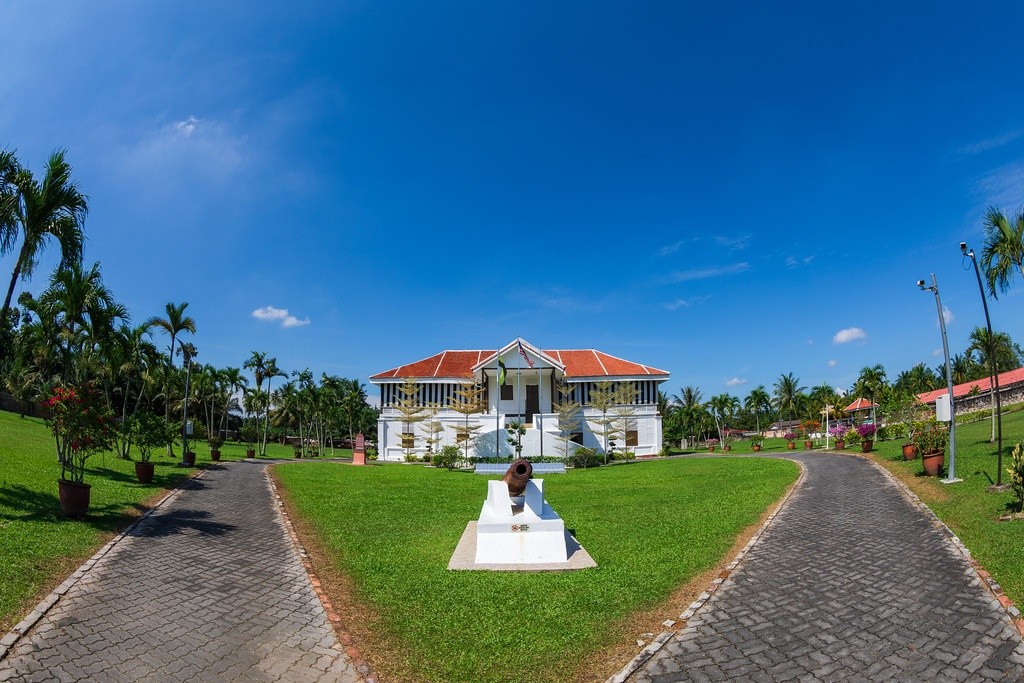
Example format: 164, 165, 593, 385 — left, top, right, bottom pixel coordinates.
708, 446, 715, 452
862, 440, 874, 453
786, 443, 795, 450
834, 440, 845, 450
56, 477, 93, 518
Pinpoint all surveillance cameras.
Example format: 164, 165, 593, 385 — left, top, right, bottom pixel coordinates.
917, 280, 934, 292
960, 242, 975, 259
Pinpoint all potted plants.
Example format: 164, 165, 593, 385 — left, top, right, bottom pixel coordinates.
880, 388, 931, 461
799, 420, 822, 449
208, 436, 224, 461
171, 415, 208, 468
750, 435, 765, 452
911, 427, 949, 478
239, 426, 263, 459
723, 437, 736, 452
291, 441, 303, 458
119, 411, 180, 484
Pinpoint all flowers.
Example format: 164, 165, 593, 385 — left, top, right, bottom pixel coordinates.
37, 382, 118, 482
856, 423, 877, 440
784, 433, 797, 442
706, 438, 718, 446
828, 425, 849, 440
